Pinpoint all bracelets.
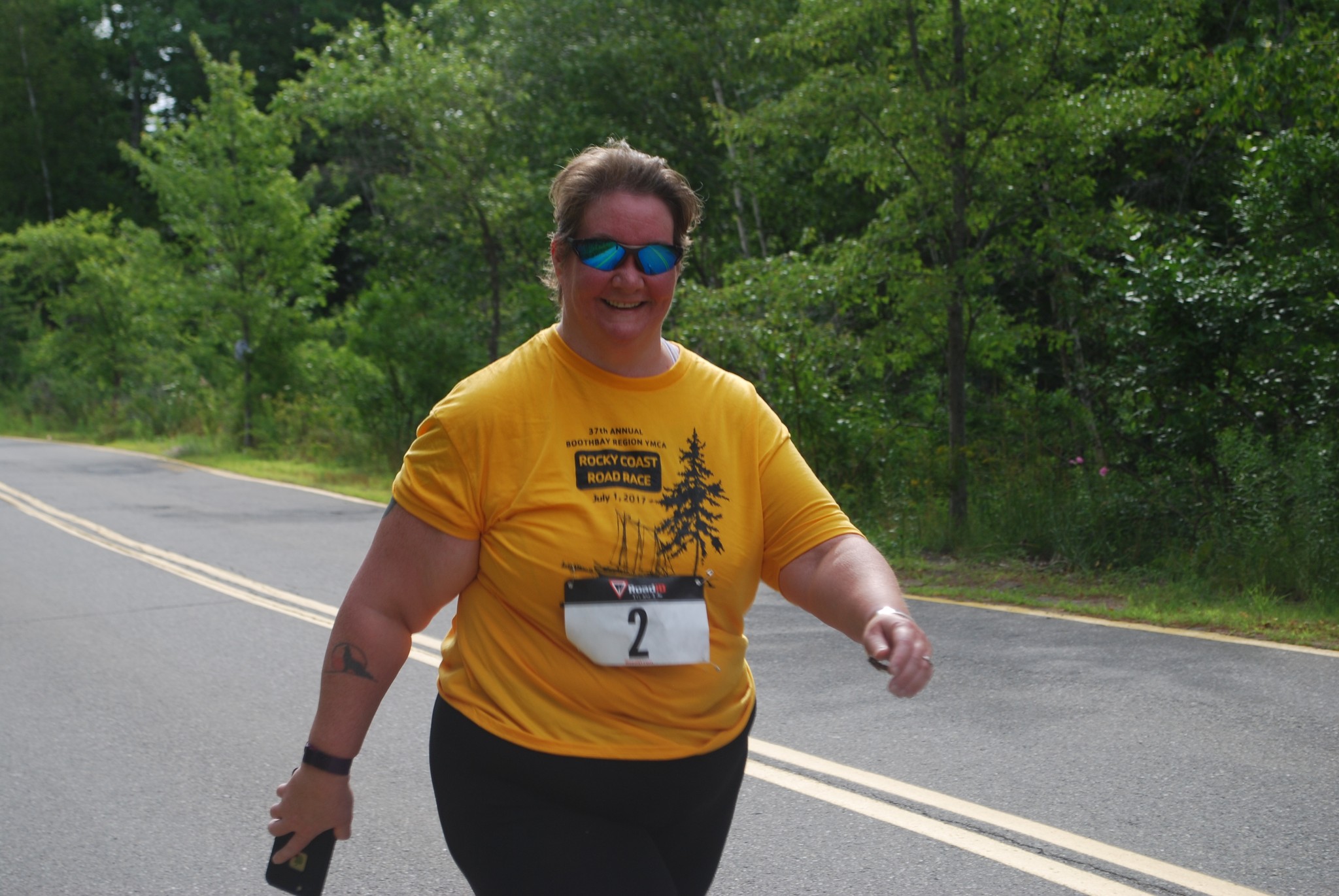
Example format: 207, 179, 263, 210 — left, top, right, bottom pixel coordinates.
302, 740, 353, 775
864, 606, 918, 673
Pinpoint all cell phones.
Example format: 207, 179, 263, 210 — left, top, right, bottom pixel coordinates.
265, 767, 336, 896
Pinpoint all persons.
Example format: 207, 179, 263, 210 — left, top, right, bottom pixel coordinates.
269, 133, 937, 896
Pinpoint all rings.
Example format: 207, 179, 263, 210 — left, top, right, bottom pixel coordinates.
923, 654, 931, 663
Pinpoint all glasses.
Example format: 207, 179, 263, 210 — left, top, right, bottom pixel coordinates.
568, 237, 684, 275
